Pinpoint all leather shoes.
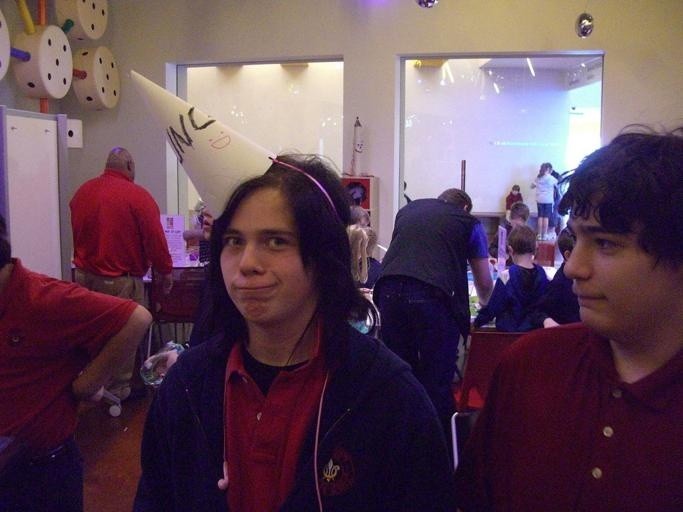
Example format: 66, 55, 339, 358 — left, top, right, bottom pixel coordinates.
121, 389, 145, 403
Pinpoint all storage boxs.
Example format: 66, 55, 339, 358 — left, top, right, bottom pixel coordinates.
533, 240, 557, 268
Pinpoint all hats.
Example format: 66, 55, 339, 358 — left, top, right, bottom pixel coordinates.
131, 69, 277, 220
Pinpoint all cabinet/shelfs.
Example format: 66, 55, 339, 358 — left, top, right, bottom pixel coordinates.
467, 211, 538, 258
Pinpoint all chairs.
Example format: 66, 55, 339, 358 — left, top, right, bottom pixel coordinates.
146, 266, 207, 347
449, 330, 543, 473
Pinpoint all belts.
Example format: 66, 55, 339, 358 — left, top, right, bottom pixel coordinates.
29, 441, 67, 467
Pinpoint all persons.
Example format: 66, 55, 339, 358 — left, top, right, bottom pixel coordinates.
354, 230, 381, 288
453, 124, 683, 512
471, 162, 581, 332
0, 214, 154, 512
373, 188, 494, 466
132, 155, 453, 512
144, 205, 215, 389
68, 148, 173, 403
350, 206, 371, 227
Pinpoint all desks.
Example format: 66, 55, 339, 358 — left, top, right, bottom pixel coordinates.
356, 287, 505, 373
70, 256, 205, 301
